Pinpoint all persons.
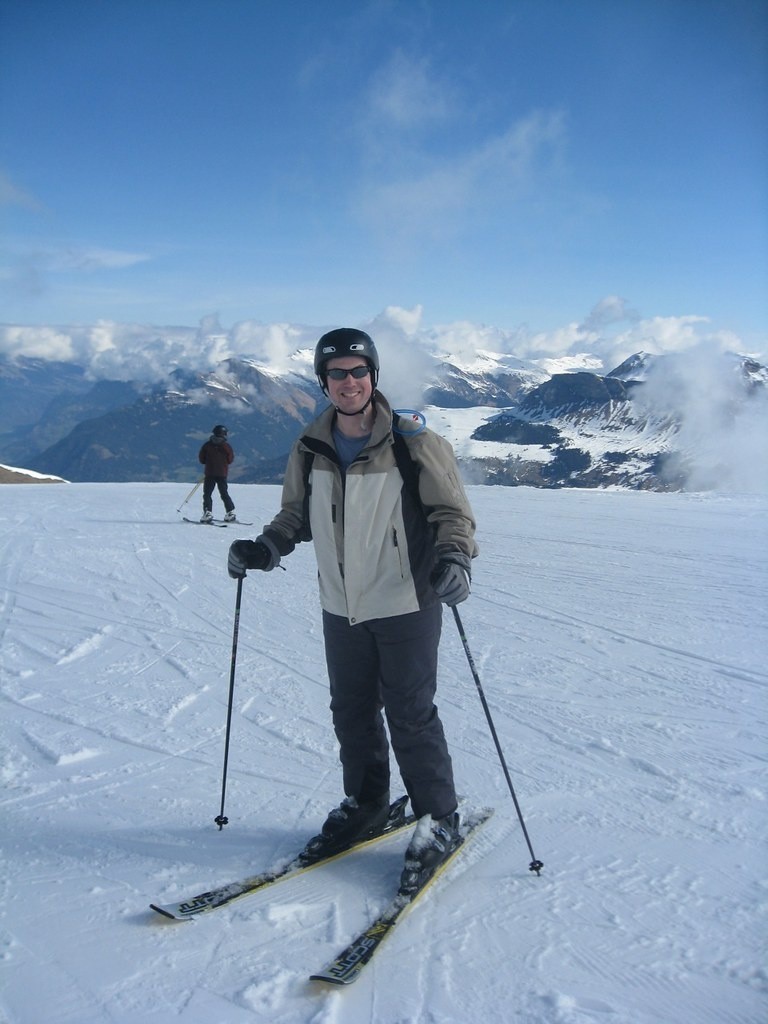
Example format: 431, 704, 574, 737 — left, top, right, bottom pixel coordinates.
228, 328, 480, 896
199, 425, 237, 525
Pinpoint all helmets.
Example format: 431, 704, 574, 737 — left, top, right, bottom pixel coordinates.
211, 425, 229, 438
314, 327, 380, 388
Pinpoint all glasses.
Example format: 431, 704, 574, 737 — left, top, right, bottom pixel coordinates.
323, 364, 373, 380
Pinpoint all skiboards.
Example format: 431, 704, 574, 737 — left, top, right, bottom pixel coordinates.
148, 790, 470, 922
306, 805, 493, 985
183, 517, 227, 528
211, 518, 253, 525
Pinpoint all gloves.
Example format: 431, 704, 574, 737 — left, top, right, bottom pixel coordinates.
227, 539, 273, 580
433, 563, 471, 608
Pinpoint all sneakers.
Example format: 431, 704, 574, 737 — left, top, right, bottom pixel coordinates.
321, 789, 390, 842
403, 814, 460, 870
224, 512, 237, 522
200, 512, 215, 524
